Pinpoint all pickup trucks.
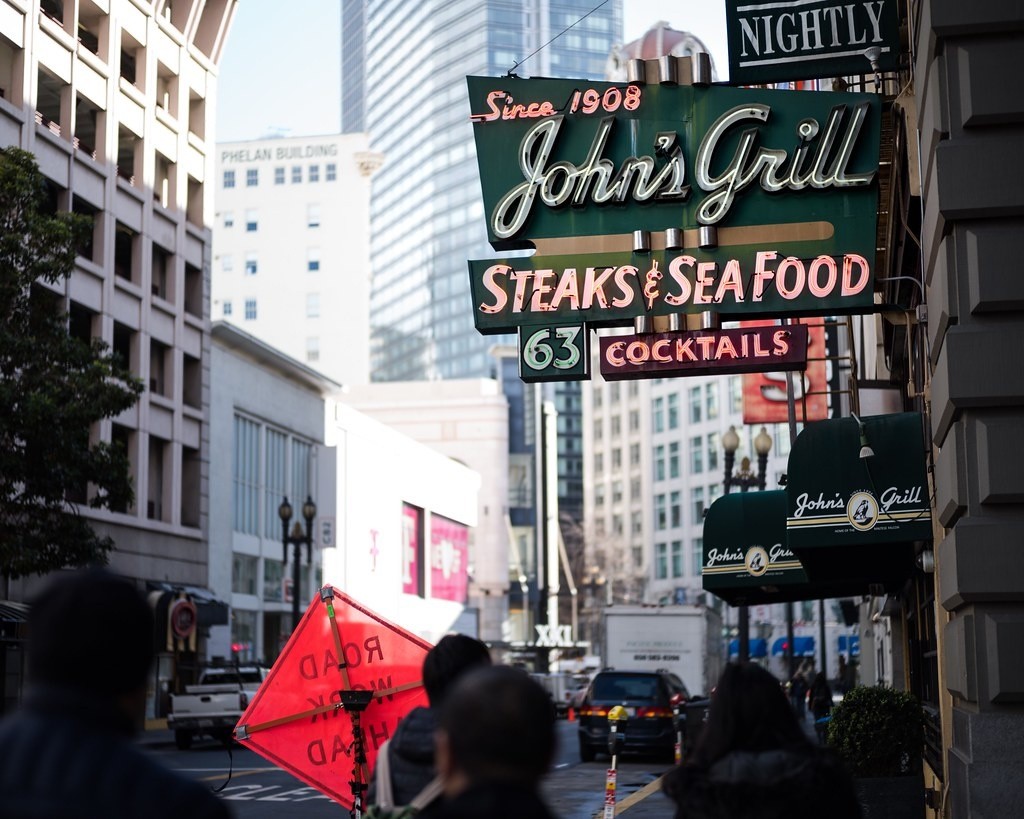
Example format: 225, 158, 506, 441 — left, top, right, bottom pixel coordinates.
170, 662, 279, 745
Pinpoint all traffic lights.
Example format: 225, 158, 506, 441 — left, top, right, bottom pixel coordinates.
782, 643, 789, 660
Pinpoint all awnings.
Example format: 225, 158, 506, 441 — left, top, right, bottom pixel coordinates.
783, 410, 933, 577
701, 488, 903, 608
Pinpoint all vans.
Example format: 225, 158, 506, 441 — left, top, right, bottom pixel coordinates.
526, 670, 578, 713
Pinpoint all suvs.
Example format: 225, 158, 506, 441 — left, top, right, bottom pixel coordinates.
577, 666, 697, 763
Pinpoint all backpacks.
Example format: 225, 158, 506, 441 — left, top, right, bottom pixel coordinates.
360, 740, 447, 819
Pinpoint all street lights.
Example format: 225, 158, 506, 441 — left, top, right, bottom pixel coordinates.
278, 494, 317, 638
722, 421, 776, 666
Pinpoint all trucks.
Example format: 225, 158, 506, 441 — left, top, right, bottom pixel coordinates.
598, 601, 727, 726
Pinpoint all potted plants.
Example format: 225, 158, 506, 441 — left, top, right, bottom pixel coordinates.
826, 681, 932, 819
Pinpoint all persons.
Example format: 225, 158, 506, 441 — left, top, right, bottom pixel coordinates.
409, 664, 567, 818
660, 660, 869, 819
787, 670, 837, 744
365, 632, 492, 819
0, 566, 244, 819
